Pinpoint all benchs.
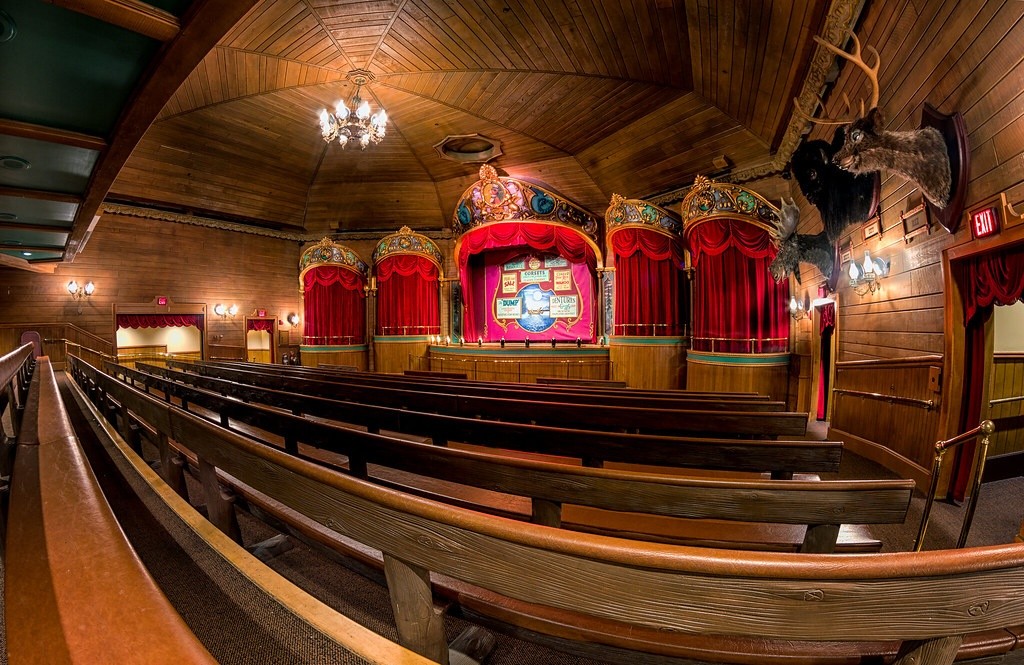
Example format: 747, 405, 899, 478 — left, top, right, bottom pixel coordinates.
0, 341, 1020, 664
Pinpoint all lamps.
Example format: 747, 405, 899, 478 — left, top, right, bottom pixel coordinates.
289, 314, 299, 327
319, 79, 388, 152
789, 292, 804, 321
214, 303, 239, 320
67, 280, 95, 315
848, 249, 889, 296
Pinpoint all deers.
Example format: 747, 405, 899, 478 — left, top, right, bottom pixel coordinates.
768, 195, 833, 284
793, 28, 951, 211
